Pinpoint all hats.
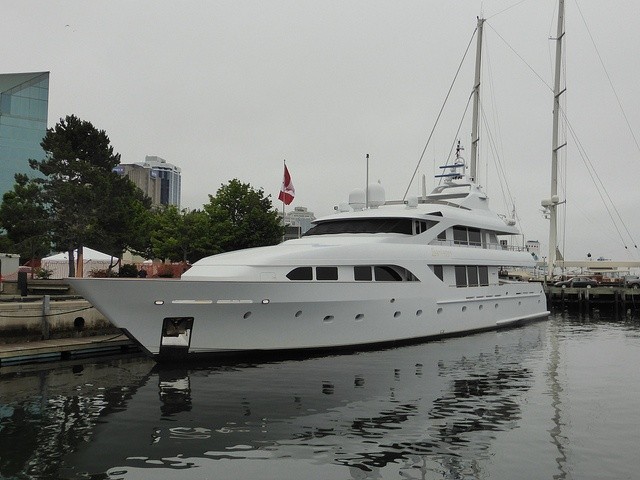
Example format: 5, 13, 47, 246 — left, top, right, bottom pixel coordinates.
554, 277, 598, 288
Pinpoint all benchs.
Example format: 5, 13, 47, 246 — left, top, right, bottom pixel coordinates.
278, 164, 295, 205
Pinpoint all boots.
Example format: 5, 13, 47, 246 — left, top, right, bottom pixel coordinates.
625, 277, 640, 289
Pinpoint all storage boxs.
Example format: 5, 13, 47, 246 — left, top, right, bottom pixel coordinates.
26, 283, 69, 294
